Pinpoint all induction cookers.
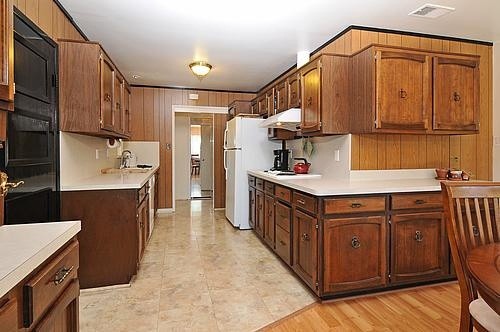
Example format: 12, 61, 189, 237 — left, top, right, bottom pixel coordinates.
267, 171, 322, 179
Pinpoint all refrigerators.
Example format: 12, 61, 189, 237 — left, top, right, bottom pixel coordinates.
223, 117, 283, 230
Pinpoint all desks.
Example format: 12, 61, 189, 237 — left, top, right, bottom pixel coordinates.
464, 241, 500, 316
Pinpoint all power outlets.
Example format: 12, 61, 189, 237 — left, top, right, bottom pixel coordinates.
96, 149, 102, 160
334, 149, 339, 161
106, 148, 110, 157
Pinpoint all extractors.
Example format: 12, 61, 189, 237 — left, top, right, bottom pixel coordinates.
258, 107, 301, 132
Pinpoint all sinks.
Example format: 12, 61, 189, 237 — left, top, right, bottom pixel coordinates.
102, 167, 149, 174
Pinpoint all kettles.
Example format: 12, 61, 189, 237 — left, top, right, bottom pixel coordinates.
292, 157, 311, 174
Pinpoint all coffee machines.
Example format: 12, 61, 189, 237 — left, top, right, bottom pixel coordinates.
270, 149, 289, 171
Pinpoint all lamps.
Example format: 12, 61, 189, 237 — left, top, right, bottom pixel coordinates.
188, 61, 212, 82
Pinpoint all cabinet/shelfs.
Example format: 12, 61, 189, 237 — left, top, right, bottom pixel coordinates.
275, 183, 293, 270
0, 0, 15, 112
56, 37, 115, 136
319, 193, 388, 300
287, 72, 300, 110
154, 171, 159, 218
450, 198, 500, 281
265, 179, 275, 252
145, 180, 152, 251
61, 185, 146, 294
115, 64, 124, 137
388, 192, 450, 290
300, 52, 349, 137
352, 42, 481, 134
0, 285, 19, 332
276, 81, 295, 140
250, 92, 266, 117
266, 87, 276, 139
293, 189, 319, 298
256, 176, 265, 241
124, 76, 134, 138
248, 174, 256, 232
19, 234, 86, 331
228, 100, 252, 118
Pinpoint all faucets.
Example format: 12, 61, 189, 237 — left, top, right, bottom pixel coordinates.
119, 149, 134, 169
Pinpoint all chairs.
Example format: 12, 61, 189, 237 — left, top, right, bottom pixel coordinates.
439, 179, 500, 332
191, 154, 201, 175
